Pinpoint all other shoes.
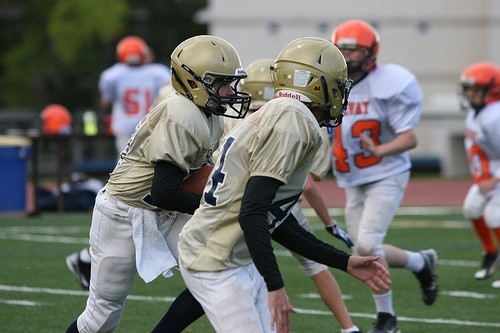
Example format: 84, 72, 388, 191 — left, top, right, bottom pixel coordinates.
474, 252, 500, 279
490, 280, 500, 288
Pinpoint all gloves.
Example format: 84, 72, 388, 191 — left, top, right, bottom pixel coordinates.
326, 220, 355, 250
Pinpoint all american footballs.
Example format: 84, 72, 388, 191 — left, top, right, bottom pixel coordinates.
183, 165, 214, 195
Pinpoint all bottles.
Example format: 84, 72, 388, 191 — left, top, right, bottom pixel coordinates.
83, 105, 98, 136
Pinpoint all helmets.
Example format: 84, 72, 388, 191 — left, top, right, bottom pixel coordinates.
271, 37, 349, 120
460, 62, 500, 104
240, 58, 276, 112
115, 35, 152, 64
40, 104, 72, 135
170, 35, 248, 109
331, 20, 381, 72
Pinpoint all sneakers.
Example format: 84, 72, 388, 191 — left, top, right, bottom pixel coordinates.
65, 248, 91, 291
412, 248, 440, 307
374, 312, 401, 333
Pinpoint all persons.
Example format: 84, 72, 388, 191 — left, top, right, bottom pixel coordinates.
177, 34, 392, 333
40, 95, 71, 134
98, 35, 172, 159
459, 64, 500, 289
323, 20, 440, 333
65, 58, 362, 333
61, 35, 252, 333
